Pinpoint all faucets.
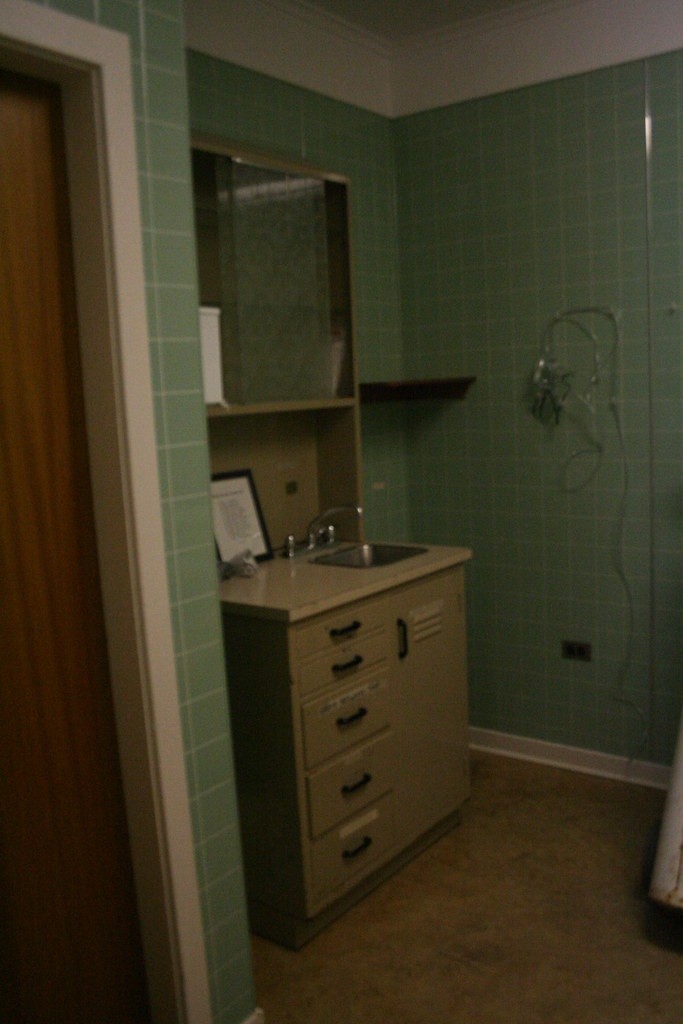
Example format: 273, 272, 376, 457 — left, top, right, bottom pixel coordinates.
306, 504, 364, 549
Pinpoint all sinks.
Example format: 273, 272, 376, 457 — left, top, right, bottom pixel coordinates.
308, 541, 430, 571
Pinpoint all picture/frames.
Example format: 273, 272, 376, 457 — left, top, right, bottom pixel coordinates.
211, 468, 275, 563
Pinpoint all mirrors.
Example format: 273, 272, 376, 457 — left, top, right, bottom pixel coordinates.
190, 148, 356, 407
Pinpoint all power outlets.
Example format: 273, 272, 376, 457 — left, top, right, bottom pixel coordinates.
562, 640, 591, 662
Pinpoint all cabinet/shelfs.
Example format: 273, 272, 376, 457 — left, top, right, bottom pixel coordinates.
221, 576, 485, 918
